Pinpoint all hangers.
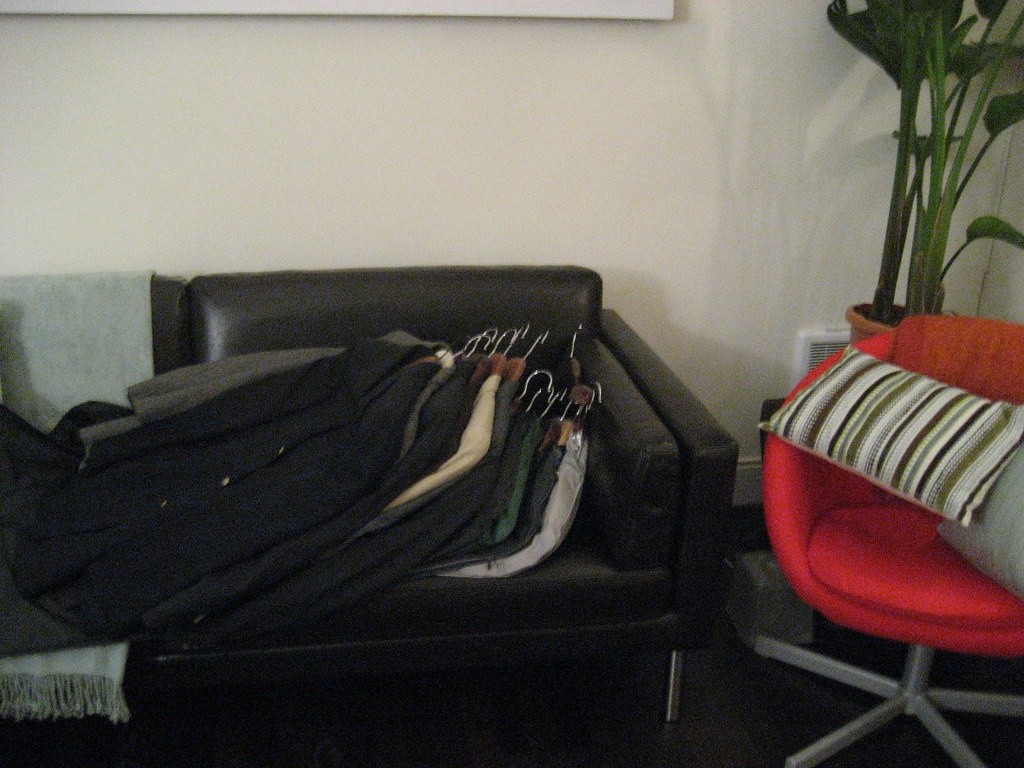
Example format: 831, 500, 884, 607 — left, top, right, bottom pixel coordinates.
430, 321, 604, 450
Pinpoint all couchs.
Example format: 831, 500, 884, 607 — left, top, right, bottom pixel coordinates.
0, 265, 741, 768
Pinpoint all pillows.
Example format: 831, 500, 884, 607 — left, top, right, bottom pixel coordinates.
937, 446, 1024, 599
756, 343, 1024, 528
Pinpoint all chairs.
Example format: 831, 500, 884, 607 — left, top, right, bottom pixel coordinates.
744, 315, 1024, 768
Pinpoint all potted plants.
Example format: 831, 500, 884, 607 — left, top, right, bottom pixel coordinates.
824, 0, 1024, 347
